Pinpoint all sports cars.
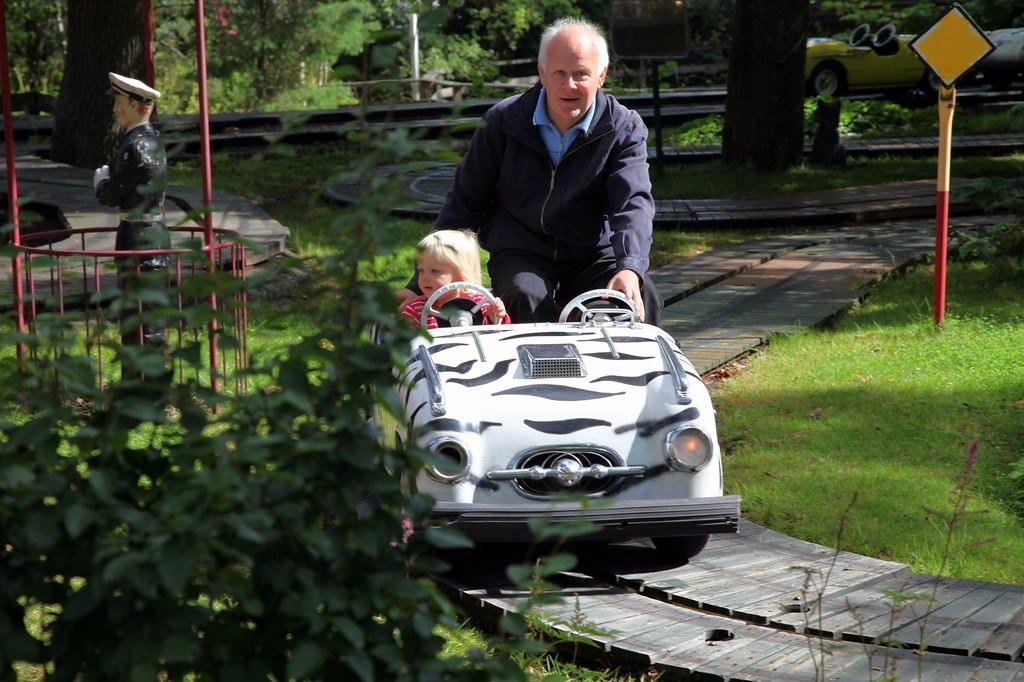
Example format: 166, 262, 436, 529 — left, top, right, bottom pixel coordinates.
805, 24, 1024, 103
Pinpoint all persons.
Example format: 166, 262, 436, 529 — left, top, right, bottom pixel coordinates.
399, 228, 511, 331
392, 14, 664, 328
86, 72, 171, 430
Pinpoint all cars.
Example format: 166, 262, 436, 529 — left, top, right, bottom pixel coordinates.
367, 280, 744, 581
